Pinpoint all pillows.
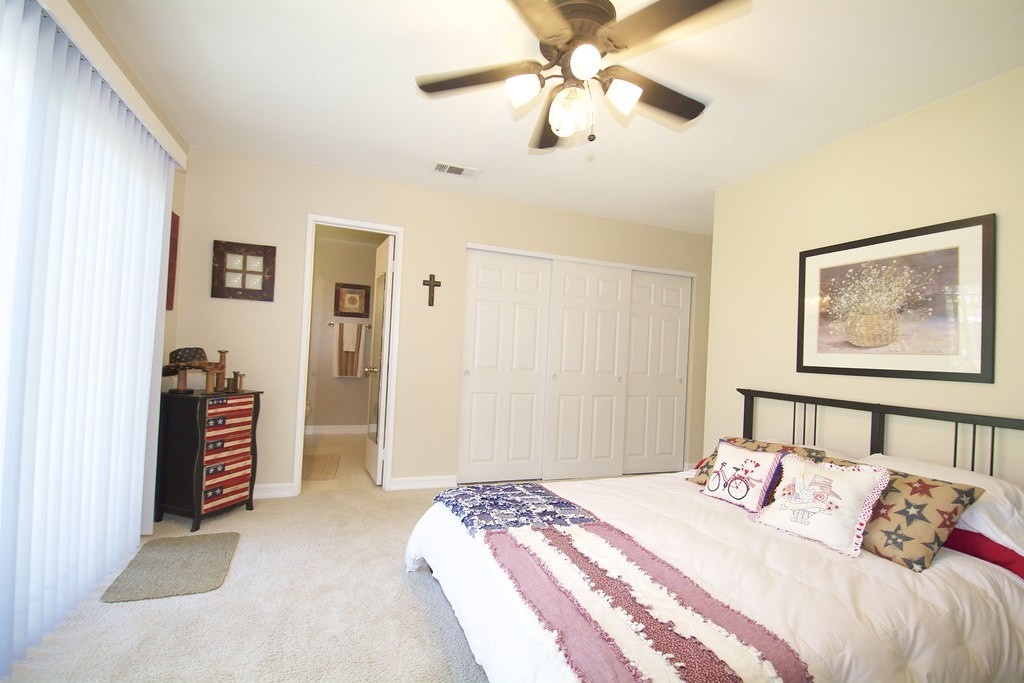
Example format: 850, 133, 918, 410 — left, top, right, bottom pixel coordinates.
699, 439, 791, 514
685, 435, 827, 506
753, 452, 891, 559
853, 453, 1024, 557
758, 439, 852, 463
817, 456, 986, 573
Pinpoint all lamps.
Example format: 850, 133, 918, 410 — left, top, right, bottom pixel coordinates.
505, 44, 644, 141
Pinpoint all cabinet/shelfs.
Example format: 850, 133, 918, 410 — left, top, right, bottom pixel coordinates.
458, 243, 695, 483
153, 389, 264, 531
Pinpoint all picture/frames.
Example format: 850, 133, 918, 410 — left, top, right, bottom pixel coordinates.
334, 283, 371, 318
796, 213, 996, 384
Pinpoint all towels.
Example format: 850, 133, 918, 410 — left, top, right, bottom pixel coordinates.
331, 322, 366, 379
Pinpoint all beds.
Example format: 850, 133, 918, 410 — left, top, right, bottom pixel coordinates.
406, 388, 1024, 683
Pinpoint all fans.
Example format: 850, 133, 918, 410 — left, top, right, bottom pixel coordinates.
413, 0, 751, 154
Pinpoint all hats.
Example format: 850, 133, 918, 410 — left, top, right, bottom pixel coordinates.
161, 348, 225, 377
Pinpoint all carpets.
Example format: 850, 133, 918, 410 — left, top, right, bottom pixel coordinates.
100, 532, 240, 603
302, 453, 340, 481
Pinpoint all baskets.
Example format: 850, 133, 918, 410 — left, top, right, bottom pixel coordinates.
846, 309, 898, 347
